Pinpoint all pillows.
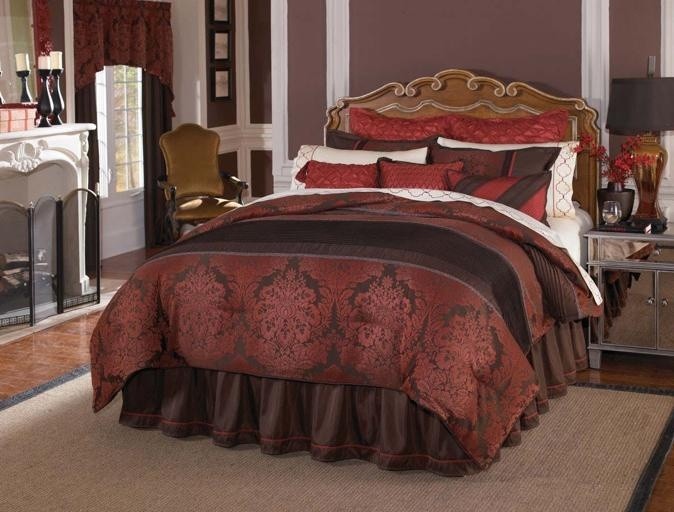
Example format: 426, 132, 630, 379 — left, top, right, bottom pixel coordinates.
290, 103, 583, 229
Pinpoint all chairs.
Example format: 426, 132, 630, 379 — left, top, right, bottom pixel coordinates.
155, 120, 251, 241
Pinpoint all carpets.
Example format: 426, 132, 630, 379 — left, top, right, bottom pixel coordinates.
0, 356, 673, 511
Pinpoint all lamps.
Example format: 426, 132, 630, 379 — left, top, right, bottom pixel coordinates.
603, 75, 673, 231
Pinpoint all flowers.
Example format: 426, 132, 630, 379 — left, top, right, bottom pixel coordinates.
572, 131, 656, 184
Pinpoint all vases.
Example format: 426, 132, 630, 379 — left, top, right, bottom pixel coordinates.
596, 181, 634, 221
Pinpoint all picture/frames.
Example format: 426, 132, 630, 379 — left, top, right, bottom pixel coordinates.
208, 29, 232, 65
208, 67, 234, 103
208, 0, 232, 25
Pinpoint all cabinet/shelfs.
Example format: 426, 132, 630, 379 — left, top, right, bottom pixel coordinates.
582, 229, 673, 370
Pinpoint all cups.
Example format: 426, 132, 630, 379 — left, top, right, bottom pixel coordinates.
601, 200, 622, 227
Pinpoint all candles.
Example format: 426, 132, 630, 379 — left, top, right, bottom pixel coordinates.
48, 50, 64, 69
14, 52, 31, 72
36, 55, 52, 71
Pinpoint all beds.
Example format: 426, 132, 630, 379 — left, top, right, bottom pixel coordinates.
85, 66, 604, 480
